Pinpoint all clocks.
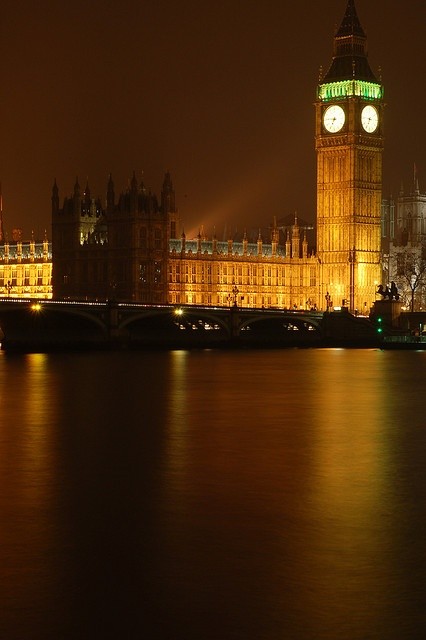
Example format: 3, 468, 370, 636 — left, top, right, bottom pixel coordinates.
322, 104, 346, 133
360, 104, 380, 134
